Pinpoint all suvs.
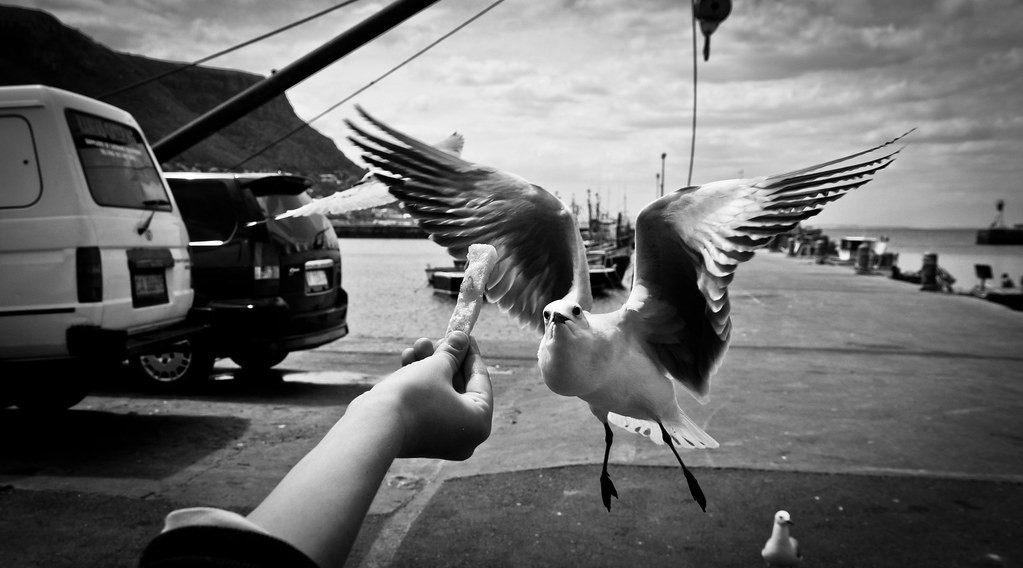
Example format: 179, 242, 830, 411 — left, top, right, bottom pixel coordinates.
130, 169, 351, 396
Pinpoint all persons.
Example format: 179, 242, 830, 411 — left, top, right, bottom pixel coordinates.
137, 329, 494, 567
766, 226, 947, 294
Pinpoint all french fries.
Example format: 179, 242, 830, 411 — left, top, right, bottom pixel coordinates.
446, 243, 498, 337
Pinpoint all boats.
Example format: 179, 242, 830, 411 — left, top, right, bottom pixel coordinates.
973, 197, 1023, 248
422, 186, 635, 297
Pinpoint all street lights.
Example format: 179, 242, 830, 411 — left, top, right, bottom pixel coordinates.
660, 153, 668, 197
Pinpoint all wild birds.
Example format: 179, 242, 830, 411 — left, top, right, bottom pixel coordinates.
344, 100, 917, 512
761, 509, 800, 561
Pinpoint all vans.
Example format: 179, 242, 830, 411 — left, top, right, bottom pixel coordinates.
0, 84, 197, 417
